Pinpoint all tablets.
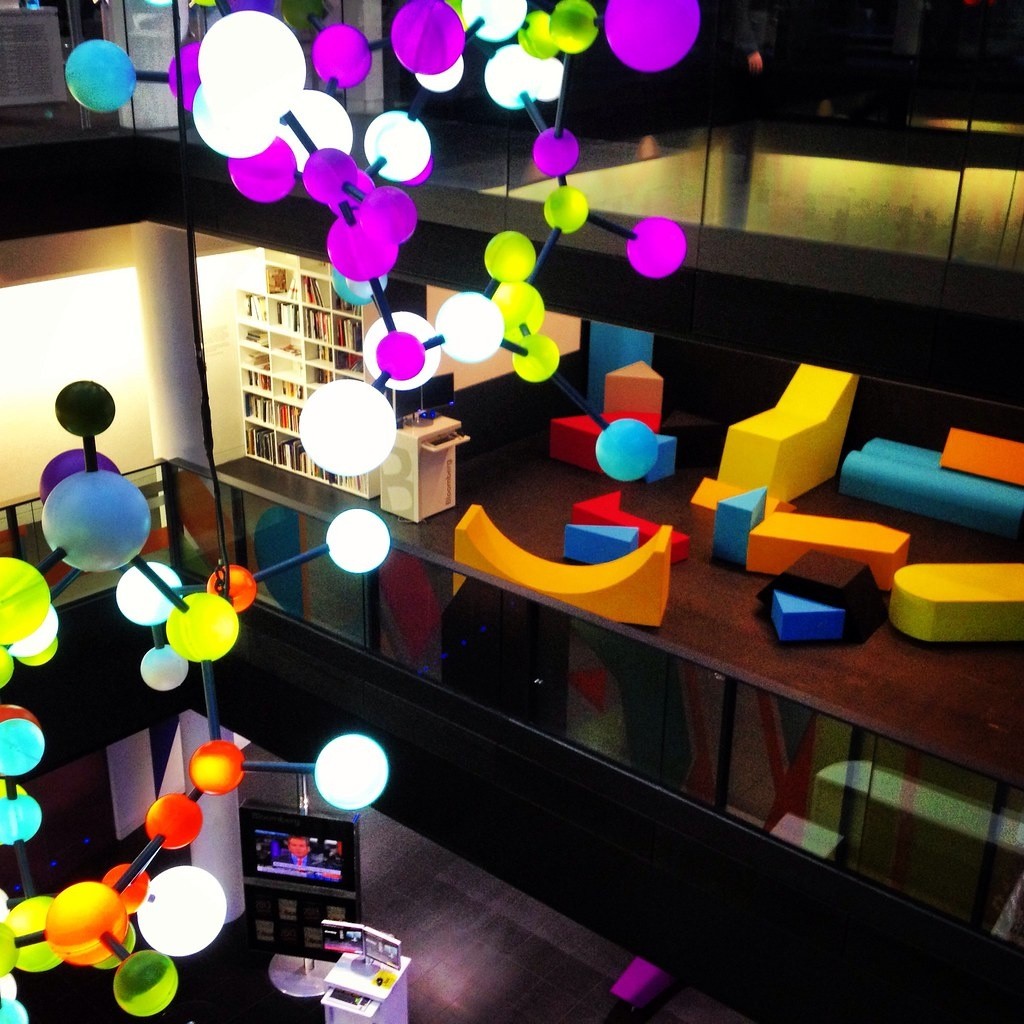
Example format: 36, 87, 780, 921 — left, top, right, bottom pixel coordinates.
239, 797, 361, 900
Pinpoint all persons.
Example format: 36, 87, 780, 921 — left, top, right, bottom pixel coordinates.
734, 0, 781, 119
280, 835, 317, 873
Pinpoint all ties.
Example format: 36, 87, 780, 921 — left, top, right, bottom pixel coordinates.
297, 859, 303, 872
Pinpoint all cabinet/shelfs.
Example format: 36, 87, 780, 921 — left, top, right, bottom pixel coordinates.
233, 248, 388, 500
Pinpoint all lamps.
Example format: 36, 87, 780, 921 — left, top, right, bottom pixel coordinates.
0, 383, 395, 1024
61, 0, 703, 480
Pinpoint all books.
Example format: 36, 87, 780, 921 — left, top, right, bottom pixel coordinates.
244, 267, 367, 492
